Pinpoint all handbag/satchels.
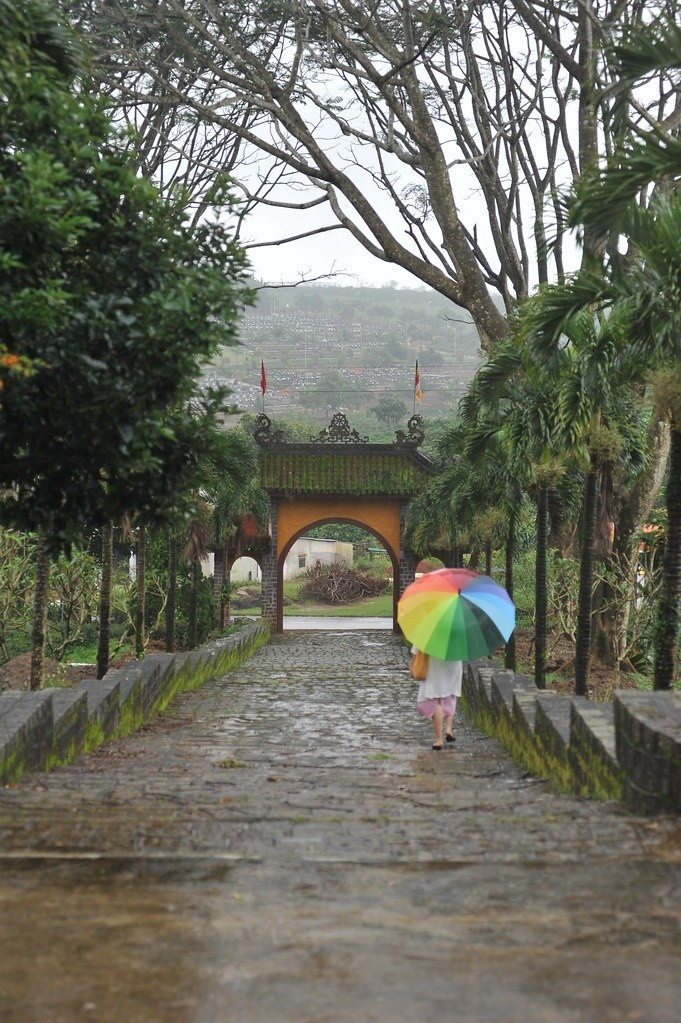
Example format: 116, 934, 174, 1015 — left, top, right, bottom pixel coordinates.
412, 651, 430, 681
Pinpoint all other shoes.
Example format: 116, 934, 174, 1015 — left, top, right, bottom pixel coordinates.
446, 733, 456, 742
433, 743, 442, 750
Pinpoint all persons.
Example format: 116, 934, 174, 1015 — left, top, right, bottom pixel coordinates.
411, 645, 463, 749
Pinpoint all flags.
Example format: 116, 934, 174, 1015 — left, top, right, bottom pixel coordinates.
261, 359, 266, 396
416, 360, 421, 401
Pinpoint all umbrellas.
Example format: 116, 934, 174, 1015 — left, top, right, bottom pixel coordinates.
397, 568, 516, 662
